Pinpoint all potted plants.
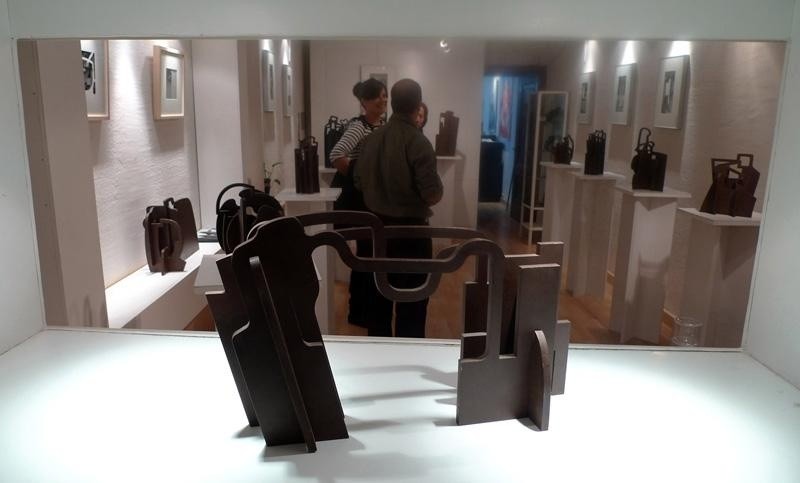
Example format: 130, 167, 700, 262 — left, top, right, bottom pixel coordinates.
264, 162, 283, 196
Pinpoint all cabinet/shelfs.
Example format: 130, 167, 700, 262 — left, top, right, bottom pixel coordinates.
519, 90, 569, 246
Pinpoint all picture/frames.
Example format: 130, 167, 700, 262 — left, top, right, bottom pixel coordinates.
576, 72, 596, 125
153, 45, 184, 120
262, 50, 276, 113
653, 54, 690, 129
360, 64, 393, 122
610, 64, 638, 126
282, 64, 294, 118
80, 39, 110, 122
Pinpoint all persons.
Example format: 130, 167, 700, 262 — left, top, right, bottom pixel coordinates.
328, 78, 388, 328
414, 101, 428, 132
350, 78, 445, 337
